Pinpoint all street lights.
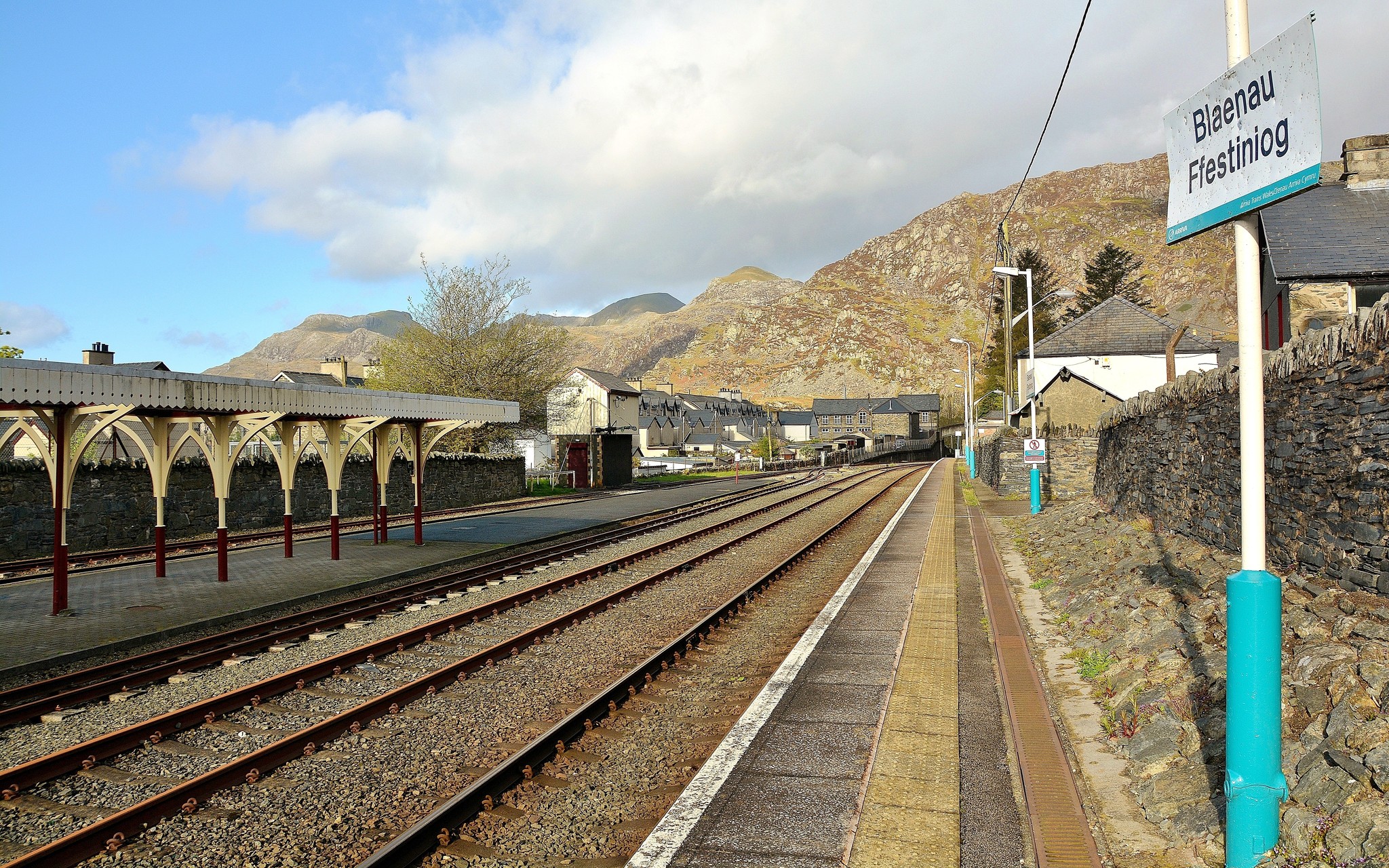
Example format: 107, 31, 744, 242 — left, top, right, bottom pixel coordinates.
948, 337, 1003, 480
993, 266, 1078, 516
